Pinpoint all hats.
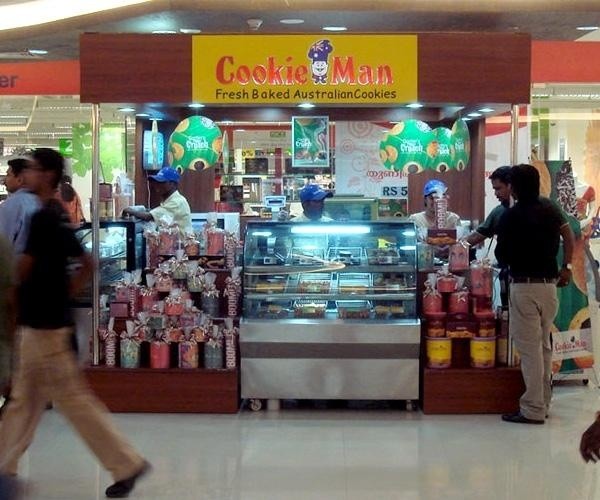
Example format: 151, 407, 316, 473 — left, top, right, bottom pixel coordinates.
423, 179, 448, 196
147, 167, 180, 183
300, 184, 333, 205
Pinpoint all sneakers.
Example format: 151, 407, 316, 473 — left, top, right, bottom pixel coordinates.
105, 460, 152, 499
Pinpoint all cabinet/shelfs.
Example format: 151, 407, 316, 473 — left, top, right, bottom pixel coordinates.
81, 239, 240, 414
416, 262, 533, 414
240, 219, 421, 414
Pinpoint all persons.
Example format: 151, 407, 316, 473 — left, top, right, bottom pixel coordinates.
405, 178, 463, 246
0, 145, 152, 499
464, 165, 520, 309
495, 165, 574, 426
0, 158, 43, 276
580, 413, 600, 465
54, 175, 85, 233
285, 185, 339, 257
121, 167, 193, 243
363, 196, 406, 220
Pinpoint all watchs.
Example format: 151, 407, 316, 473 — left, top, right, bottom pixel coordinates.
562, 263, 573, 270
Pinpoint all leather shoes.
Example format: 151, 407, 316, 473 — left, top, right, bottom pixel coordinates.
502, 411, 545, 424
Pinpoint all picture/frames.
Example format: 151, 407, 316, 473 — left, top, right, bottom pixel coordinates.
291, 116, 330, 169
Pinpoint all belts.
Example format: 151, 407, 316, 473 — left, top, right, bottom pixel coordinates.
508, 277, 555, 283
